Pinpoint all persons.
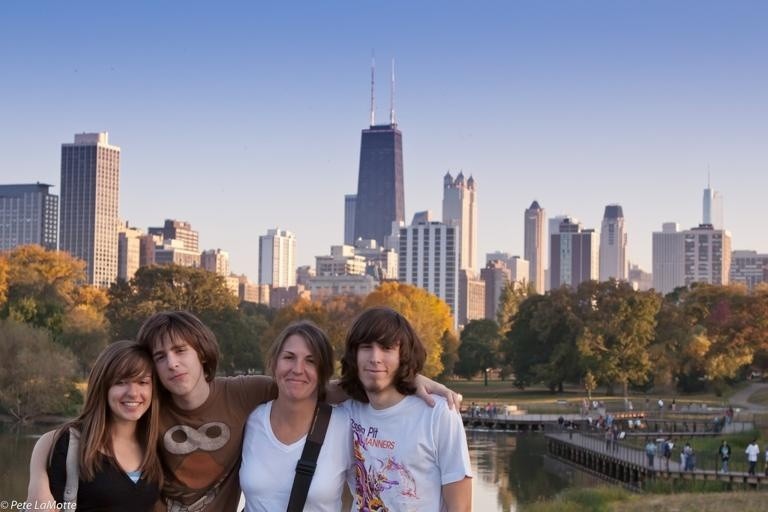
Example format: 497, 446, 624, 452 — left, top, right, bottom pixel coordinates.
25, 311, 462, 512
558, 396, 768, 477
46, 340, 167, 510
470, 401, 500, 418
239, 323, 359, 512
336, 304, 474, 511
